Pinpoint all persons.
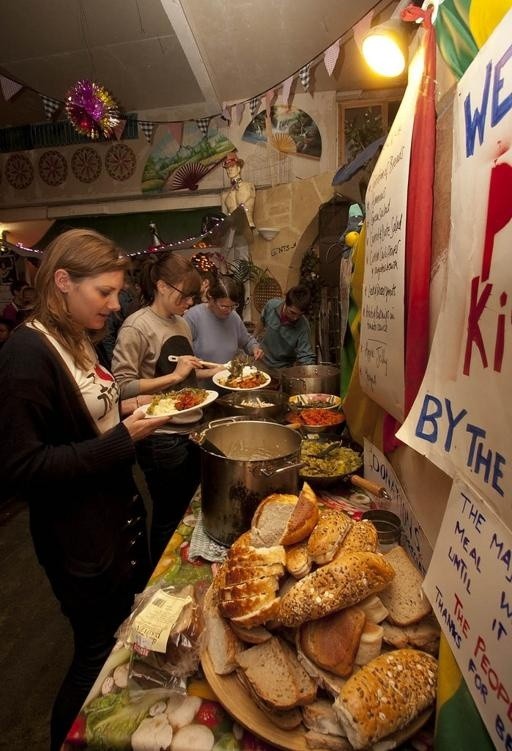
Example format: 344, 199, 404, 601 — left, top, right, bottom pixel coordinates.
182, 275, 264, 397
0, 228, 173, 751
3, 281, 29, 342
252, 285, 317, 372
111, 251, 227, 569
220, 153, 256, 228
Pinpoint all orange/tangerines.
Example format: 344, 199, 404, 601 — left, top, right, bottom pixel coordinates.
345, 231, 359, 246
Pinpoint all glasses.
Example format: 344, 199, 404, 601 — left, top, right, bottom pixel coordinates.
167, 283, 197, 301
215, 300, 241, 311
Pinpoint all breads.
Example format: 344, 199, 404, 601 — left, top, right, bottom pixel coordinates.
204, 482, 439, 750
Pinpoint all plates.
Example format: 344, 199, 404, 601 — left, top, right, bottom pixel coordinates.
213, 369, 271, 392
133, 389, 220, 420
167, 410, 203, 425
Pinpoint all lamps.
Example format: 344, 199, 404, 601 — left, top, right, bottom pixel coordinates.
360, 0, 412, 78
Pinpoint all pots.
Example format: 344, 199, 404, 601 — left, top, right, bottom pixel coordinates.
299, 434, 390, 506
197, 417, 308, 551
284, 364, 340, 395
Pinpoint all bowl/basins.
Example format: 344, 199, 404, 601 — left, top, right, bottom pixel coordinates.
220, 390, 345, 432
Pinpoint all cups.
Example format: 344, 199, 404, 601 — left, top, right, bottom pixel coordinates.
360, 510, 402, 545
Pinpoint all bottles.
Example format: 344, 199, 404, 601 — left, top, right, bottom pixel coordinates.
347, 203, 363, 232
